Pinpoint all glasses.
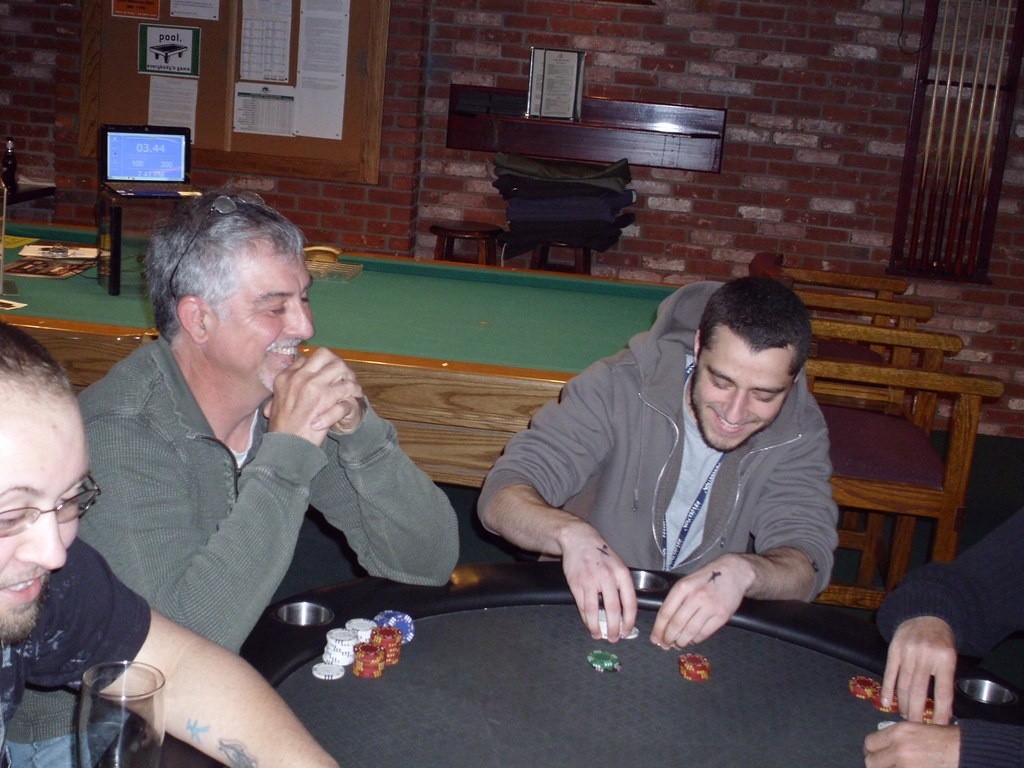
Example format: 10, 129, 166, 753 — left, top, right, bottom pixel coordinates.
0, 474, 102, 538
169, 190, 265, 301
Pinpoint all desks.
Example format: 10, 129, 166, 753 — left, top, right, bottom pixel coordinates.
0, 220, 680, 487
98, 557, 1024, 768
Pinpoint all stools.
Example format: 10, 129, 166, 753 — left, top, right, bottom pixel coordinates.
429, 222, 504, 267
529, 235, 592, 275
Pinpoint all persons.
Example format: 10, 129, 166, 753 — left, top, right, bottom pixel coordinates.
0, 320, 338, 768
476, 275, 839, 650
863, 505, 1024, 768
4, 190, 460, 768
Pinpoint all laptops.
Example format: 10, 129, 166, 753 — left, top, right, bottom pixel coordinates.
99, 121, 206, 199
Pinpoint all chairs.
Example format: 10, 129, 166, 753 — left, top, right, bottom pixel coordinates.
747, 252, 1004, 608
